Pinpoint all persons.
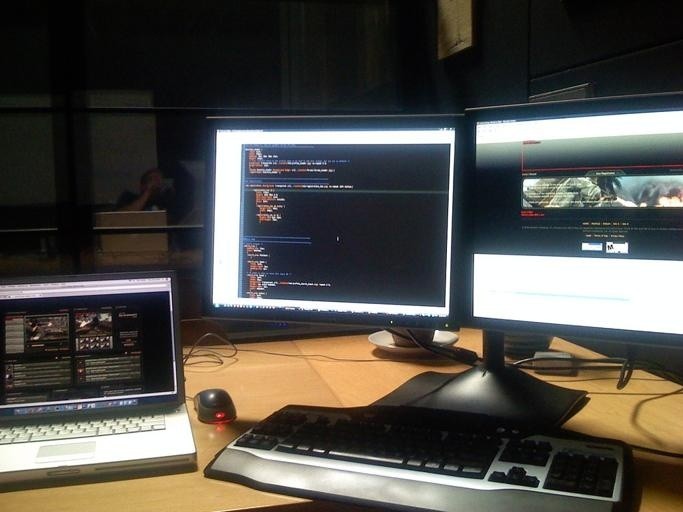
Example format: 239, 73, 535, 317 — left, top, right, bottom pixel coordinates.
110, 167, 194, 214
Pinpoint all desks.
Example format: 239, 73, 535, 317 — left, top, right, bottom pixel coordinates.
0, 323, 683, 512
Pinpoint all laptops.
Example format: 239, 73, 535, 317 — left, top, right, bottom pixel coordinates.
0, 269, 198, 485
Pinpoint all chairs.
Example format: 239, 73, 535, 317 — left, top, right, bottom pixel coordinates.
1, 210, 171, 276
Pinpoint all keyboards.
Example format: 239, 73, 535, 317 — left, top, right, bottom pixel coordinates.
204, 403, 642, 511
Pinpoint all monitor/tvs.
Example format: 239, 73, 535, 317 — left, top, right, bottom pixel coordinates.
201, 114, 589, 424
366, 92, 683, 423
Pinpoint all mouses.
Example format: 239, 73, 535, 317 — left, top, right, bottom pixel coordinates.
193, 388, 237, 423
